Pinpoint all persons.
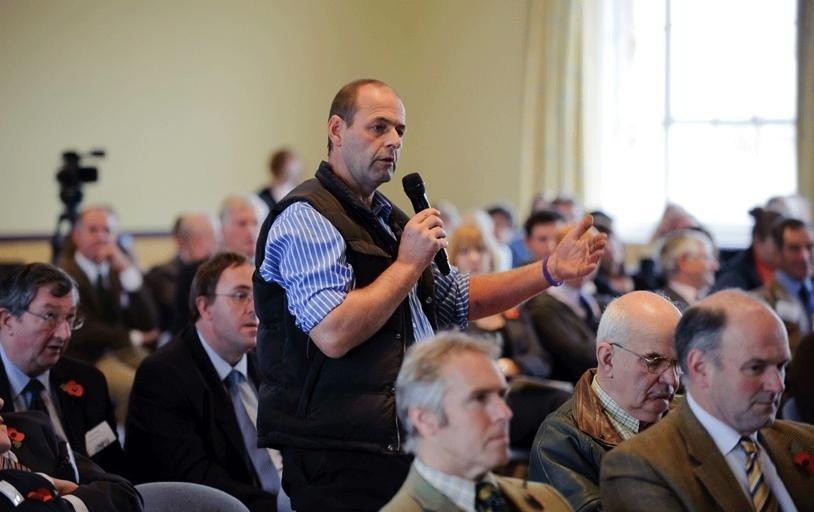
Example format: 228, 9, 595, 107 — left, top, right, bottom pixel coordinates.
255, 148, 303, 206
431, 195, 813, 512
376, 333, 575, 512
0, 196, 293, 512
248, 79, 609, 512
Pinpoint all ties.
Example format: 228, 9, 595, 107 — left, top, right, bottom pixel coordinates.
476, 482, 523, 512
739, 436, 782, 512
26, 378, 48, 416
221, 370, 280, 496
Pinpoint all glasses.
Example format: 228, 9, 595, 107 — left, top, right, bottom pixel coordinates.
24, 310, 85, 331
206, 292, 254, 305
609, 343, 685, 375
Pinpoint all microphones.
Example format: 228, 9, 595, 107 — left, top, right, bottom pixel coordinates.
400, 172, 453, 277
